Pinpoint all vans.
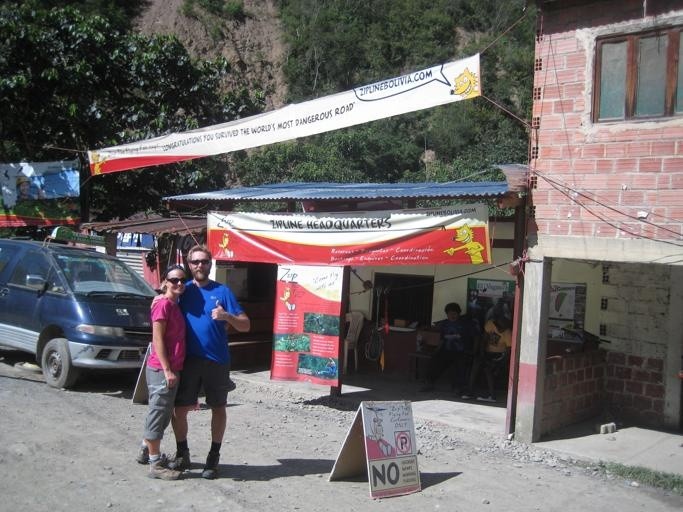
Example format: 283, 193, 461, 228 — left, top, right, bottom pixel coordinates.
0, 239, 159, 389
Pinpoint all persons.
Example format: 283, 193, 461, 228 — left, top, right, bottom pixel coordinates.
13, 176, 45, 218
137, 262, 185, 481
150, 244, 250, 480
431, 301, 512, 402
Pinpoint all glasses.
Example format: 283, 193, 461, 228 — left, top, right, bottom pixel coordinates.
191, 260, 209, 264
168, 278, 184, 283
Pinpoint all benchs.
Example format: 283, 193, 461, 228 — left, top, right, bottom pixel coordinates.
227, 297, 273, 373
409, 330, 510, 393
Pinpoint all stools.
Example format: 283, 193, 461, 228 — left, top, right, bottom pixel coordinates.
342, 310, 363, 369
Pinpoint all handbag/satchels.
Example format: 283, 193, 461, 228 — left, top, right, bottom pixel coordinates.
480, 351, 505, 369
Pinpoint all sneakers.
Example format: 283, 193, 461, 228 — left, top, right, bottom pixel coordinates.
457, 387, 496, 402
202, 457, 219, 479
135, 446, 191, 479
